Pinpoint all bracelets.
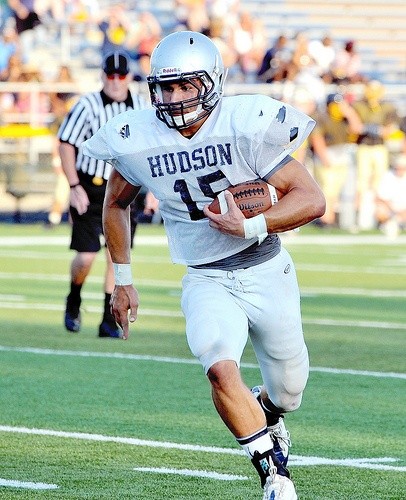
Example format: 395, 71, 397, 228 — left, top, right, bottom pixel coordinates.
69, 182, 81, 188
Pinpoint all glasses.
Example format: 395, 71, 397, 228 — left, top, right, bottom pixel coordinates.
106, 74, 126, 80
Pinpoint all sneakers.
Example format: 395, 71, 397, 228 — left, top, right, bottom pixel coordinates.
262, 473, 298, 500
250, 385, 290, 468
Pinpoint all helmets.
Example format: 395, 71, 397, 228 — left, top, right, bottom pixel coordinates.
146, 31, 229, 131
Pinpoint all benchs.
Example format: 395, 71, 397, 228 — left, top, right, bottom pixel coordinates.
250, 9, 406, 85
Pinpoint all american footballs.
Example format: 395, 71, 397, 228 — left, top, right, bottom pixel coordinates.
206, 180, 287, 222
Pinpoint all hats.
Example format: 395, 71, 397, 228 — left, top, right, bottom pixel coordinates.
102, 51, 131, 75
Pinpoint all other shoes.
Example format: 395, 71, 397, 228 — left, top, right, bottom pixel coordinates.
99, 321, 124, 338
66, 310, 81, 332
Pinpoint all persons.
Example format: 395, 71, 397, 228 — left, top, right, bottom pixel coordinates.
80, 31, 325, 500
2, 0, 262, 114
258, 32, 406, 238
57, 48, 158, 340
